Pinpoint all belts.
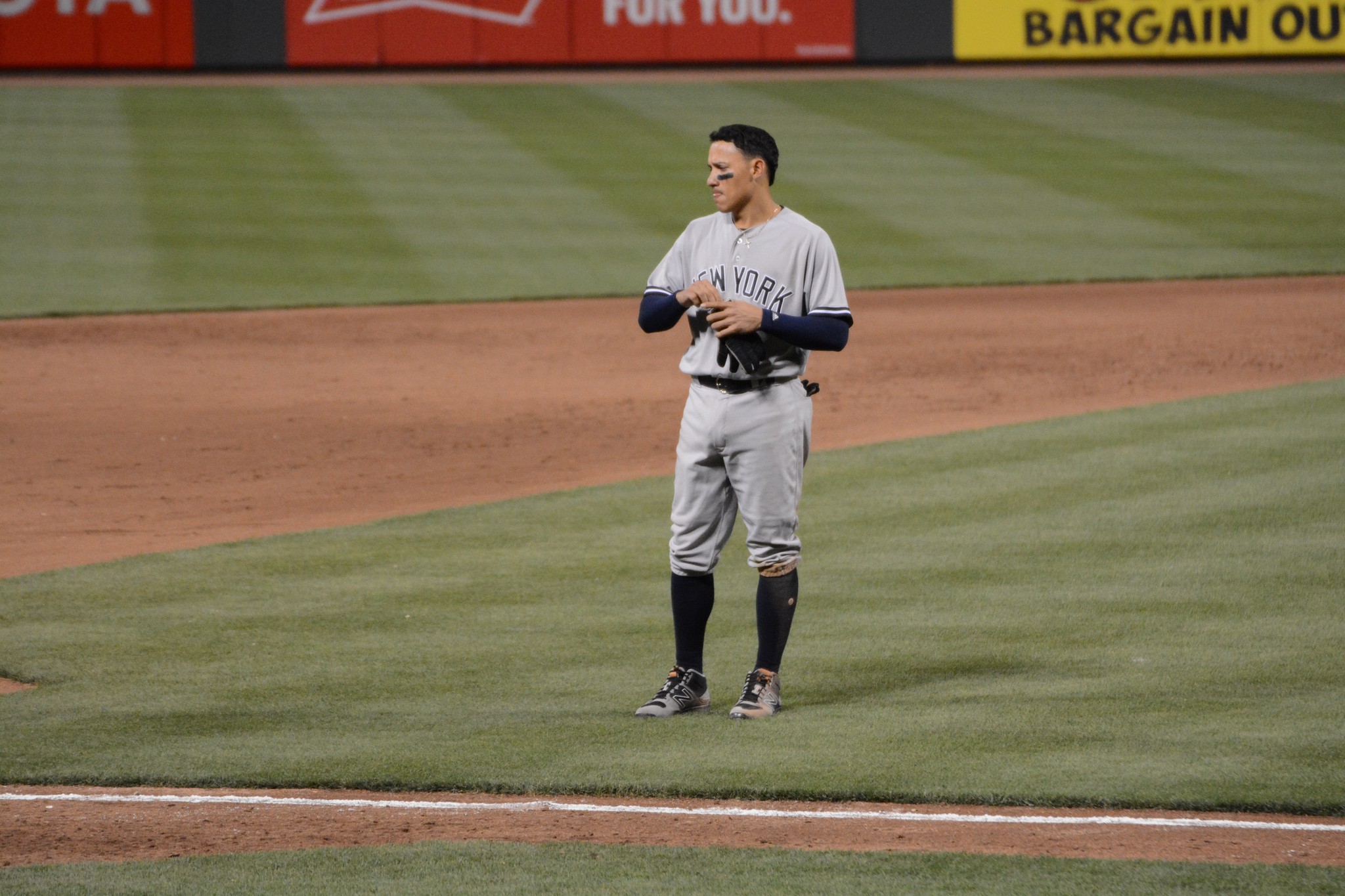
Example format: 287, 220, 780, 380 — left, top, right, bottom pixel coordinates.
698, 375, 775, 395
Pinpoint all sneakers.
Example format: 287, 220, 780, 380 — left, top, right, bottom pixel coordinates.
634, 664, 710, 717
730, 668, 783, 720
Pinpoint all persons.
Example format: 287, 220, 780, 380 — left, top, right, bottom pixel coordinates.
632, 124, 854, 719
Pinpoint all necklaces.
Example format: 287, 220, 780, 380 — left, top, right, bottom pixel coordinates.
731, 204, 780, 245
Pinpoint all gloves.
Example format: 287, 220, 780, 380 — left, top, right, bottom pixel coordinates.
801, 379, 821, 396
709, 301, 768, 374
